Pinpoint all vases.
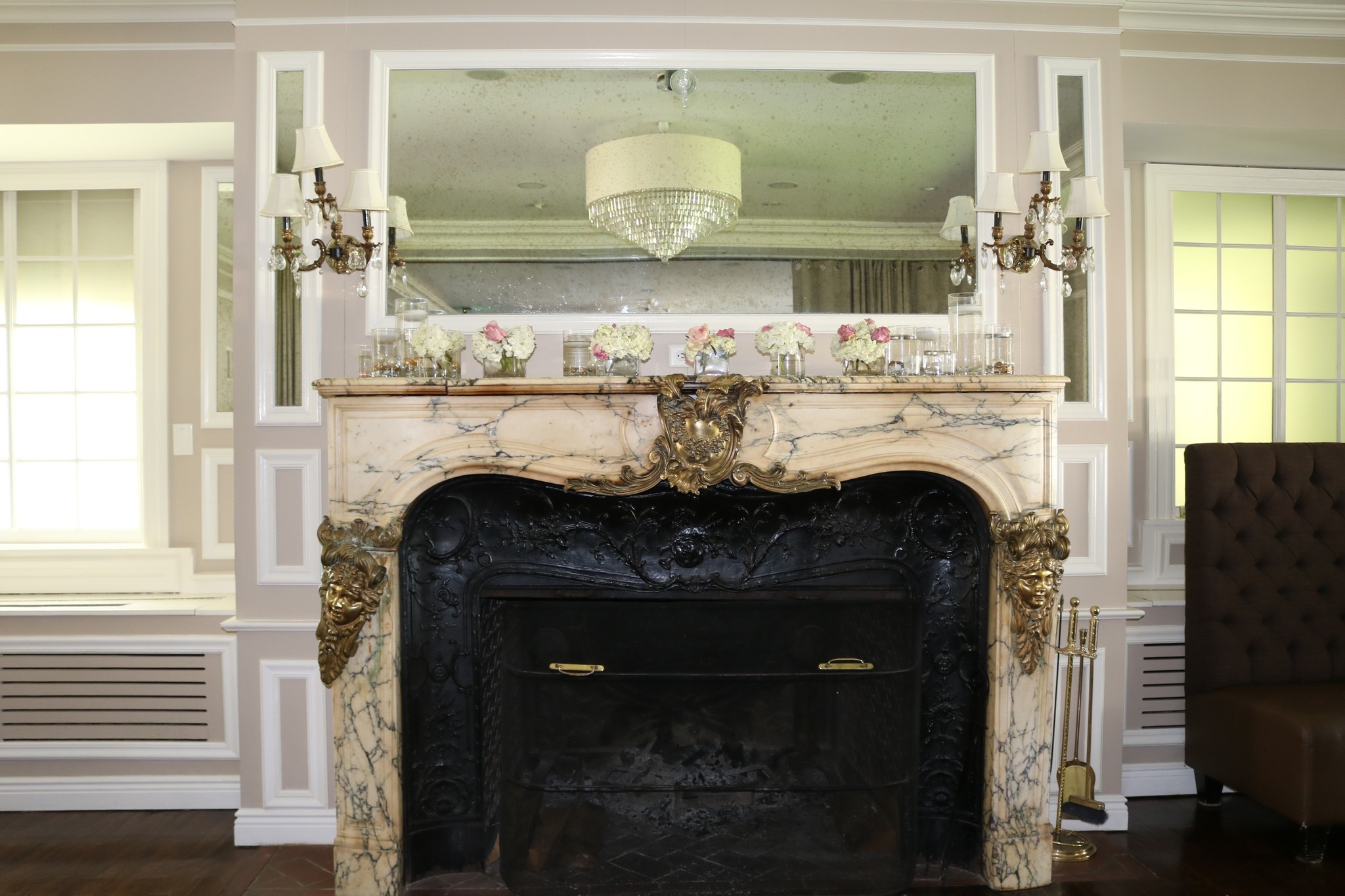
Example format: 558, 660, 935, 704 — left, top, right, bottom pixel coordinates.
694, 348, 730, 377
842, 349, 888, 376
769, 347, 805, 377
603, 356, 639, 377
482, 350, 526, 378
423, 350, 460, 378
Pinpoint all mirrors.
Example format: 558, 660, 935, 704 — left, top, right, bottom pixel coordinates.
367, 49, 996, 333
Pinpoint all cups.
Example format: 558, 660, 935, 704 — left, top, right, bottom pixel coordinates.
358, 297, 428, 377
887, 292, 1014, 376
427, 310, 446, 315
563, 330, 595, 375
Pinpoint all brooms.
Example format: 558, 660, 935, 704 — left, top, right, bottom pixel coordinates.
1061, 605, 1109, 827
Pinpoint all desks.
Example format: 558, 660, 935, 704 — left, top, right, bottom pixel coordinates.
314, 374, 1072, 896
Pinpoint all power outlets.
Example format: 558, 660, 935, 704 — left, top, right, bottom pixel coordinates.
666, 344, 694, 367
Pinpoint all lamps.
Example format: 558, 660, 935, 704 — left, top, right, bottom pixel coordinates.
259, 123, 390, 298
388, 196, 414, 286
586, 121, 742, 263
939, 196, 976, 287
973, 130, 1110, 296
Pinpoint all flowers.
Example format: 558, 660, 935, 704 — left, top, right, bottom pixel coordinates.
830, 318, 890, 371
471, 320, 536, 374
685, 323, 737, 365
588, 323, 655, 372
409, 323, 465, 358
754, 320, 814, 369
